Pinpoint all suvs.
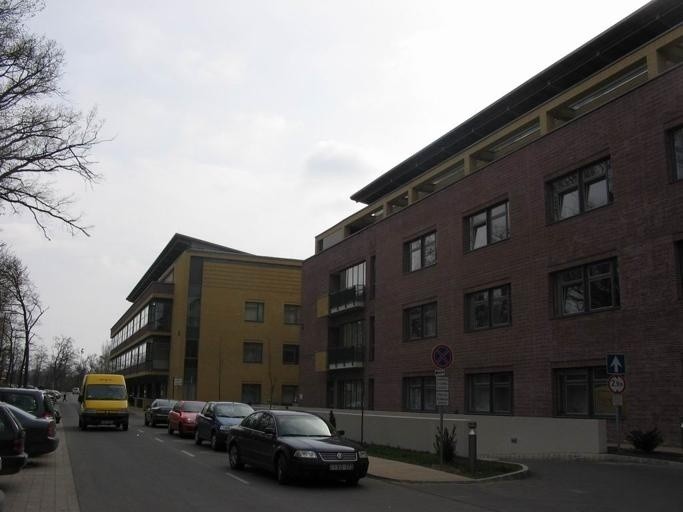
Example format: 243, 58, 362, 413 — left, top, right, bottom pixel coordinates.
0, 402, 28, 474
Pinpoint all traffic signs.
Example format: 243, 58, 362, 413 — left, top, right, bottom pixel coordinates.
435, 376, 449, 391
435, 391, 449, 406
611, 391, 624, 405
432, 369, 445, 377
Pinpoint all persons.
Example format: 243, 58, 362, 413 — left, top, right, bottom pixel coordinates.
62, 392, 68, 402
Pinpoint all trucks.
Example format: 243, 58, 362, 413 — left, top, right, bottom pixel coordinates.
78, 374, 133, 431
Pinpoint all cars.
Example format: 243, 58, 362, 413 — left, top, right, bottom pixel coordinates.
142, 399, 177, 427
228, 409, 369, 486
168, 399, 206, 438
72, 387, 80, 394
194, 401, 256, 452
0, 384, 63, 456
